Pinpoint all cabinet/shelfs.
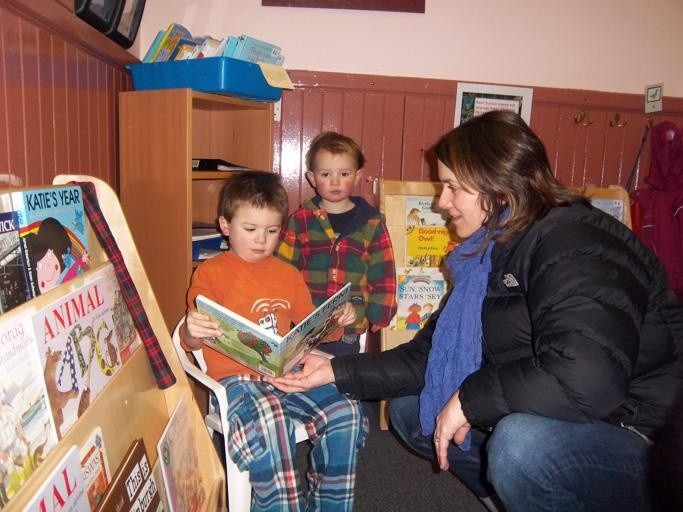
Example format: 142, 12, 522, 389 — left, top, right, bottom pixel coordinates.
368, 177, 633, 431
118, 87, 274, 422
0, 175, 226, 512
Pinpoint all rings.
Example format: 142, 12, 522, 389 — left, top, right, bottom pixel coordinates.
433, 434, 439, 442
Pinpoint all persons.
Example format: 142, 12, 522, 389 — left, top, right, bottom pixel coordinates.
258, 109, 683, 512
272, 130, 396, 361
176, 169, 368, 511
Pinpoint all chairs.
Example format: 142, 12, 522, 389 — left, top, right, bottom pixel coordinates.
172, 315, 335, 512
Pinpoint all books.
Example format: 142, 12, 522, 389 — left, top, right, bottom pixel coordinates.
195, 279, 352, 379
589, 197, 625, 226
1, 186, 218, 512
404, 196, 454, 266
138, 21, 284, 68
387, 264, 448, 333
